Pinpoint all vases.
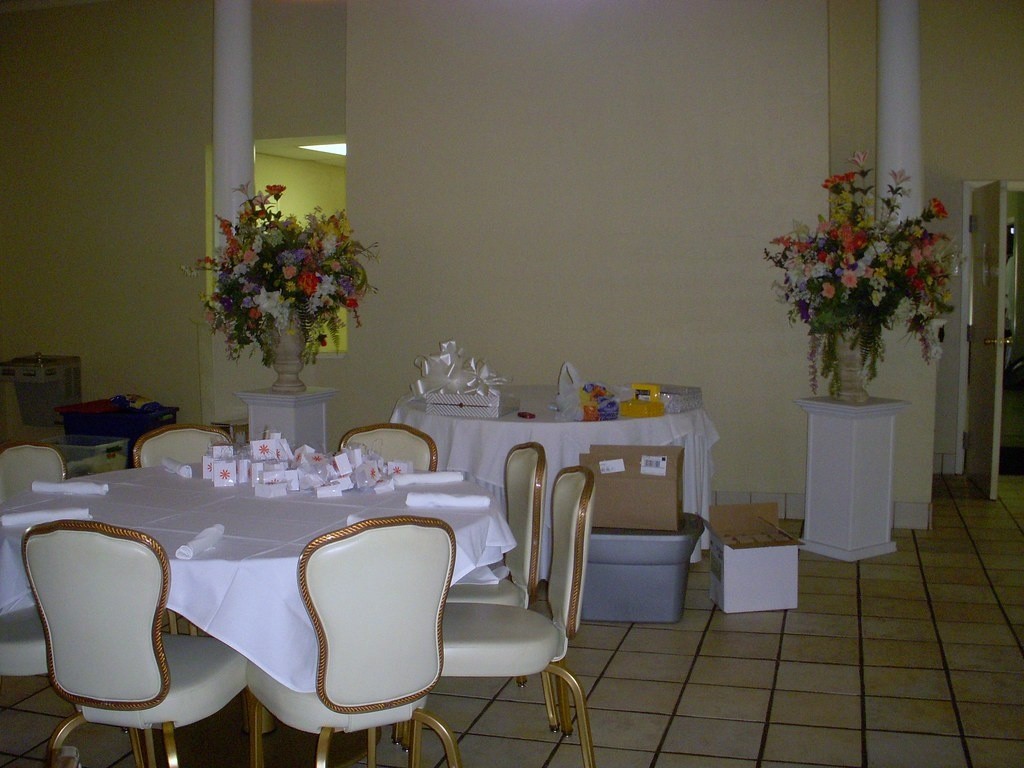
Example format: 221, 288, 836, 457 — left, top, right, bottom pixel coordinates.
261, 312, 306, 391
828, 326, 869, 403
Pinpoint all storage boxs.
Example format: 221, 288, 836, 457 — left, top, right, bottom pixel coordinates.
54, 398, 181, 466
637, 388, 702, 412
579, 443, 685, 534
426, 391, 520, 418
706, 501, 798, 613
582, 514, 704, 623
40, 436, 128, 476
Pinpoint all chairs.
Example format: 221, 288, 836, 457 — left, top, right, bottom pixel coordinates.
393, 465, 596, 768
245, 516, 461, 768
133, 424, 232, 637
440, 440, 547, 688
19, 519, 263, 768
340, 423, 437, 472
0, 439, 68, 505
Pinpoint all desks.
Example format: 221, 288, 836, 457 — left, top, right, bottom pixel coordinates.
795, 396, 909, 560
390, 384, 720, 584
233, 387, 335, 449
0, 469, 518, 768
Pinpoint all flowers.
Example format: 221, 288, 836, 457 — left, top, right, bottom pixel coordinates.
759, 146, 958, 386
179, 178, 380, 369
